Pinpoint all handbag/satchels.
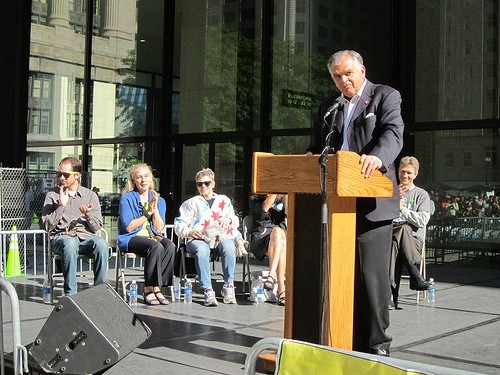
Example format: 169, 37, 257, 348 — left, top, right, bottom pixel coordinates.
249, 274, 278, 302
174, 252, 197, 280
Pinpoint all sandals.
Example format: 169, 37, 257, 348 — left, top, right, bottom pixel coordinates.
264, 276, 276, 291
142, 288, 170, 305
277, 288, 286, 306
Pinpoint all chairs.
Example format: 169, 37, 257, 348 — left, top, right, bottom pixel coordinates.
400, 225, 427, 304
115, 217, 145, 300
48, 226, 94, 305
242, 215, 269, 293
178, 238, 250, 301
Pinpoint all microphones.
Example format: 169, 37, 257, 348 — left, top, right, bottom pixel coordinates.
324, 97, 346, 118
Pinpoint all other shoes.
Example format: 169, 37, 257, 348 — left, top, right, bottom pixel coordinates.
410, 276, 434, 291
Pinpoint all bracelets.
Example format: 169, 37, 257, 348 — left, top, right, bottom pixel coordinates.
144, 213, 149, 219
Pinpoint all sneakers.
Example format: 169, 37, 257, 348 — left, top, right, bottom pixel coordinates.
204, 289, 218, 306
221, 285, 236, 304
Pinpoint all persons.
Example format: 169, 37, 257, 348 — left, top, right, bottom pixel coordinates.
117, 163, 175, 305
425, 187, 500, 227
250, 192, 286, 305
21, 174, 101, 230
309, 50, 404, 358
389, 156, 435, 311
174, 167, 239, 307
42, 157, 110, 296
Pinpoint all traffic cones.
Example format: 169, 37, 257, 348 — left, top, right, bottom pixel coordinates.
5, 225, 29, 277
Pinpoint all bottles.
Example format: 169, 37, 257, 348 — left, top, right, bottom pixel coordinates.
426, 278, 435, 304
256, 276, 263, 305
129, 280, 137, 307
184, 279, 192, 304
43, 275, 51, 304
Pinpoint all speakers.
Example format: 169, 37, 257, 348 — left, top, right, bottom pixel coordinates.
28, 282, 153, 375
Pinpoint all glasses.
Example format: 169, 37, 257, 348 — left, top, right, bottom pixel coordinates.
196, 181, 212, 187
56, 172, 78, 178
